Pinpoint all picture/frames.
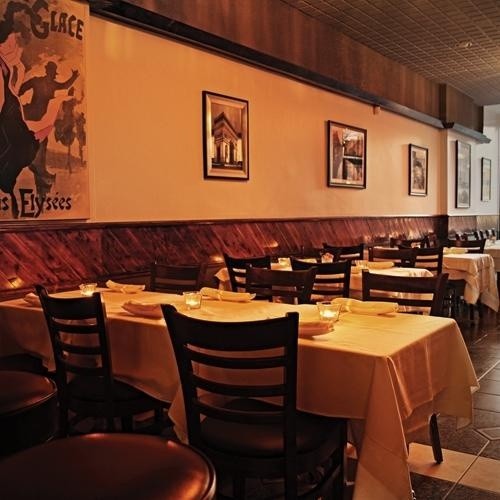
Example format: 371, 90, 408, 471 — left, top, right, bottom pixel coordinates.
407, 144, 429, 198
326, 119, 369, 192
481, 156, 492, 202
201, 87, 251, 183
455, 139, 471, 211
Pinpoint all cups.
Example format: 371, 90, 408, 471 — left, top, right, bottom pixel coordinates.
276, 258, 291, 269
318, 302, 342, 323
182, 292, 202, 309
355, 259, 367, 275
80, 283, 97, 297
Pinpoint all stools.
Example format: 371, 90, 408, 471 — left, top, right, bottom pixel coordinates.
2, 369, 58, 460
2, 432, 218, 500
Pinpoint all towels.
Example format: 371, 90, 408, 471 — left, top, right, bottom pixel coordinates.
22, 292, 164, 319
106, 280, 146, 293
198, 286, 258, 302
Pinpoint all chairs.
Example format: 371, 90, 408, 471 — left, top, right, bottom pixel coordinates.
148, 260, 205, 298
213, 227, 500, 347
37, 283, 167, 439
159, 303, 351, 499
361, 266, 451, 466
